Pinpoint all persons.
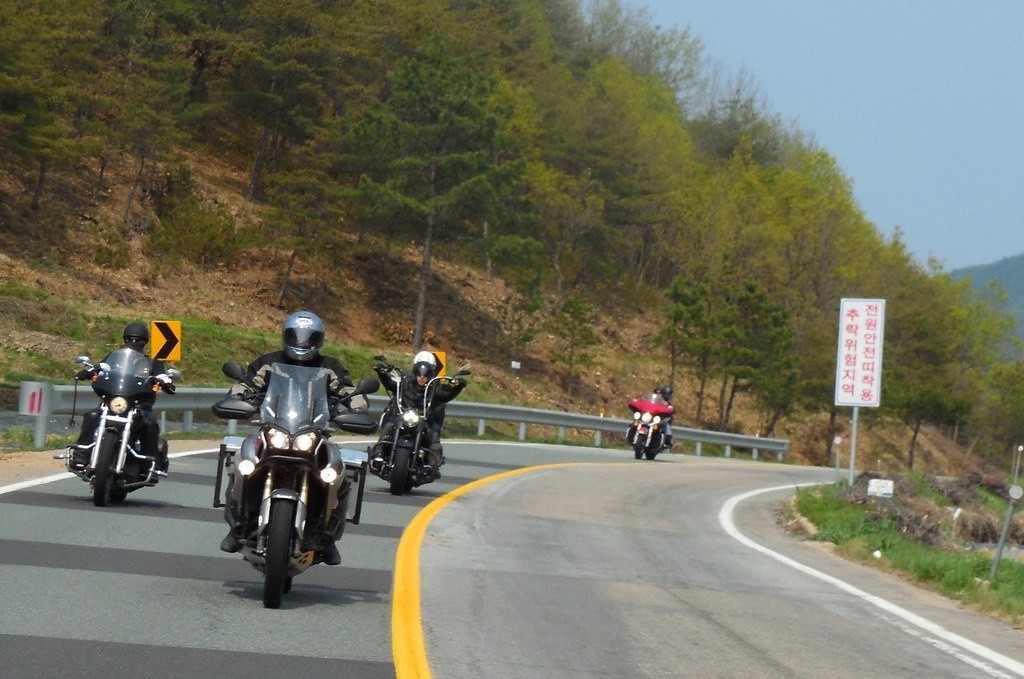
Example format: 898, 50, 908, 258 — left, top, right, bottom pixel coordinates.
626, 386, 676, 446
216, 311, 370, 566
68, 321, 173, 484
369, 351, 467, 479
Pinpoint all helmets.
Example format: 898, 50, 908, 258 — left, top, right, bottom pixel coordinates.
123, 324, 149, 345
663, 386, 673, 394
412, 361, 433, 377
413, 350, 436, 366
282, 311, 324, 362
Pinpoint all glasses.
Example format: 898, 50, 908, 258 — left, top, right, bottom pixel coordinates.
126, 337, 145, 343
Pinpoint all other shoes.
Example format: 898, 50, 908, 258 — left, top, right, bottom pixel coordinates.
71, 458, 85, 470
146, 470, 159, 484
665, 434, 672, 446
221, 529, 241, 553
323, 542, 341, 565
427, 465, 442, 479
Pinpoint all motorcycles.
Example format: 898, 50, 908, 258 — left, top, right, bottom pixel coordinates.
210, 361, 382, 610
50, 355, 186, 507
370, 353, 473, 497
625, 392, 677, 462
